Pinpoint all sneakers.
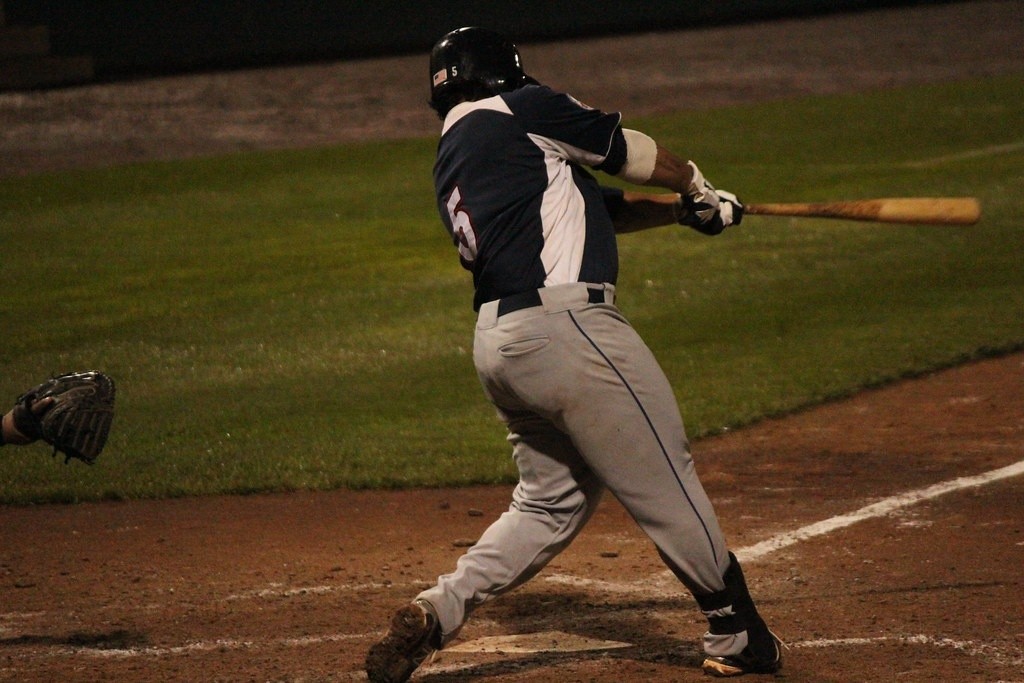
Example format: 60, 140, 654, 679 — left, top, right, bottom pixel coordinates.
700, 627, 789, 676
365, 599, 445, 683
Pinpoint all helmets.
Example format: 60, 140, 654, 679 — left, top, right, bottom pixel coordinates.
429, 27, 524, 102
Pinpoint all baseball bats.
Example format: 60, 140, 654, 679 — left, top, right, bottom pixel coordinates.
672, 196, 981, 226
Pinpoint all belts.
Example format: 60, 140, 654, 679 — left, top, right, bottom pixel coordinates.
497, 287, 616, 317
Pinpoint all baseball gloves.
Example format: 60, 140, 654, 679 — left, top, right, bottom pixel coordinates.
14, 369, 117, 466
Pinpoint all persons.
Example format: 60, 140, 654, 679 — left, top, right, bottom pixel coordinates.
0, 370, 115, 464
366, 28, 782, 683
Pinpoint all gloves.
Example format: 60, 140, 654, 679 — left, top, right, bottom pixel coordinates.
673, 190, 743, 236
677, 158, 720, 225
